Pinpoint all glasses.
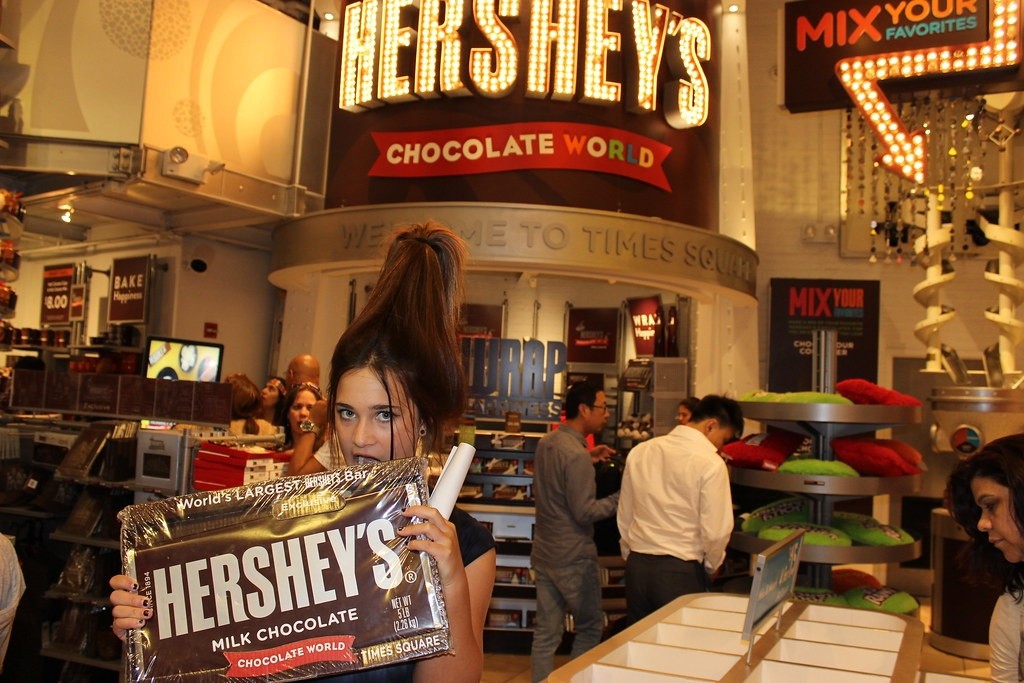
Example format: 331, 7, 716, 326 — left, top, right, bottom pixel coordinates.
293, 382, 321, 393
586, 404, 608, 416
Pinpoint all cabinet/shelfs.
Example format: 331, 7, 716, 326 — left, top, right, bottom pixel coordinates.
36, 461, 148, 679
423, 430, 627, 655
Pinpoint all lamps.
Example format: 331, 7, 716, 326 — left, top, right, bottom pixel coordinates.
54, 195, 78, 223
161, 147, 227, 184
802, 222, 838, 244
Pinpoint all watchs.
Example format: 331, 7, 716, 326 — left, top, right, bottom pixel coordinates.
300, 420, 320, 436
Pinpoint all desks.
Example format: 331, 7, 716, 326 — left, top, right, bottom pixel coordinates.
539, 593, 926, 683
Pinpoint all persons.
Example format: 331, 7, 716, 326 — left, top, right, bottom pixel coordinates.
280, 382, 326, 452
285, 354, 321, 391
675, 397, 701, 424
257, 376, 286, 428
225, 371, 260, 434
530, 381, 621, 683
617, 394, 744, 625
109, 218, 497, 683
947, 433, 1024, 683
287, 400, 347, 476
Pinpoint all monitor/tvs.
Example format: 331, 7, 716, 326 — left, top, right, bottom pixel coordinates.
141, 336, 224, 383
